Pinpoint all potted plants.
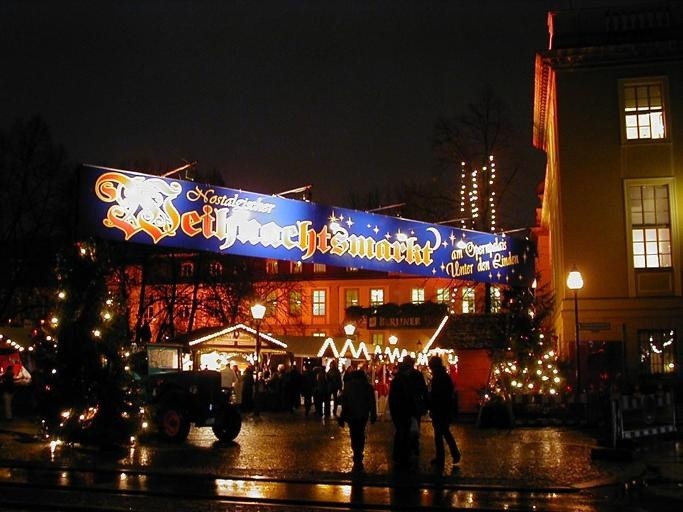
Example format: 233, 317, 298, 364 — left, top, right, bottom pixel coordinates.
345, 303, 447, 325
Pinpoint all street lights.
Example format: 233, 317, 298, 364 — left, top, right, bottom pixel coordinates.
249, 304, 266, 419
565, 271, 583, 424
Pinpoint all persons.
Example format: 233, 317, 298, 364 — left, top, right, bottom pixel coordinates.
2, 366, 16, 421
28, 359, 47, 423
220, 356, 458, 419
338, 370, 377, 477
600, 368, 632, 427
388, 356, 430, 460
426, 357, 460, 466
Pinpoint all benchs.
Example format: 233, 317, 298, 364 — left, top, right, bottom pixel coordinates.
610, 387, 680, 449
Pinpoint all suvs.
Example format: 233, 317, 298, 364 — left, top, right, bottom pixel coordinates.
131, 342, 241, 446
